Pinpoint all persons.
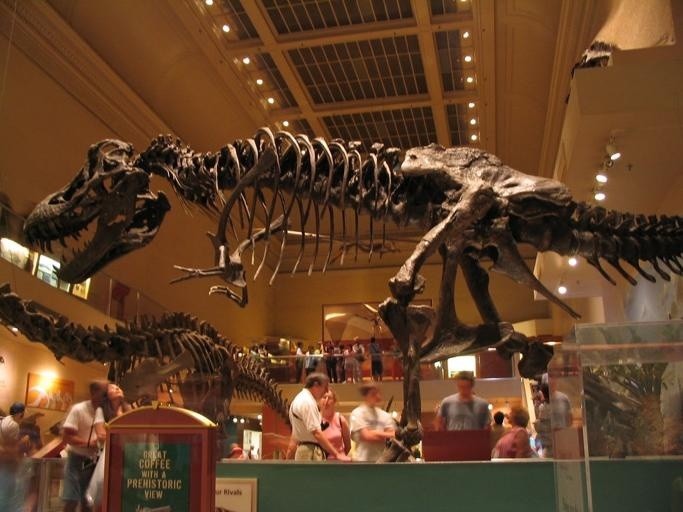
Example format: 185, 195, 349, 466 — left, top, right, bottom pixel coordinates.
349, 381, 401, 461
434, 372, 493, 432
287, 373, 353, 462
319, 385, 351, 460
491, 370, 572, 458
0, 378, 132, 512
253, 336, 402, 382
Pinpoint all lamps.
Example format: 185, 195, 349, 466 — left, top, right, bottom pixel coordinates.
590, 135, 621, 203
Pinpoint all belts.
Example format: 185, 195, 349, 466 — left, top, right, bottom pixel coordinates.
295, 441, 320, 448
